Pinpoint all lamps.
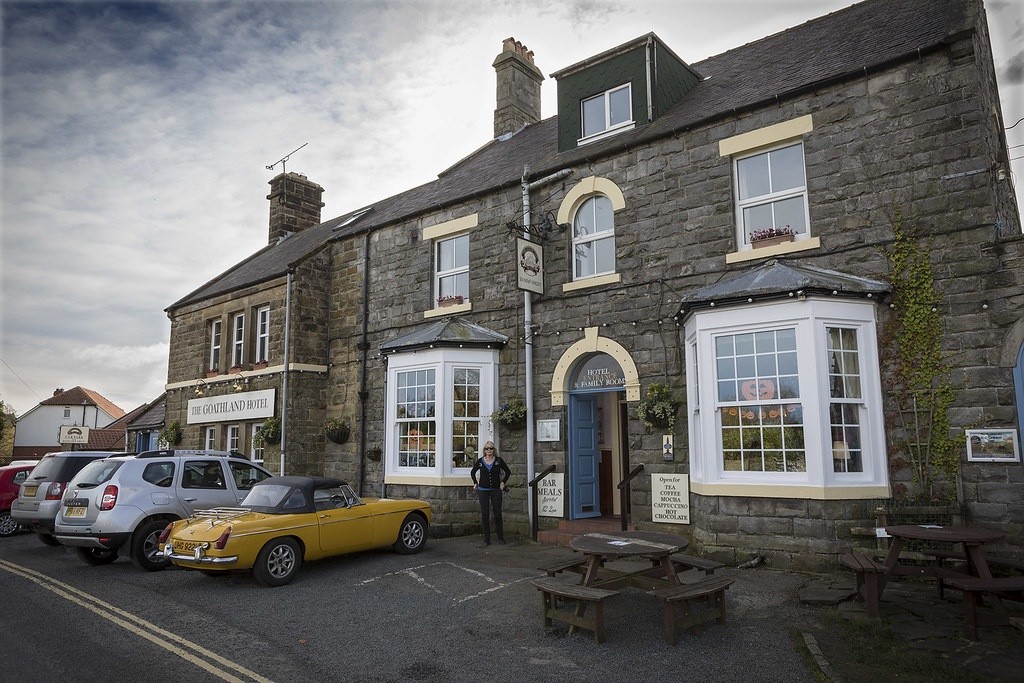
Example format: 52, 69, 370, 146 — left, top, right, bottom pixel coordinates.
233, 372, 249, 389
195, 379, 211, 394
540, 212, 568, 233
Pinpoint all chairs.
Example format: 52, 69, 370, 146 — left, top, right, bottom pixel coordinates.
285, 492, 306, 509
184, 469, 192, 487
201, 466, 220, 488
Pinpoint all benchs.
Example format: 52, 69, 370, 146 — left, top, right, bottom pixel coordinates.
922, 551, 1024, 590
536, 556, 607, 609
646, 574, 734, 646
841, 552, 889, 616
641, 555, 724, 576
944, 577, 1024, 641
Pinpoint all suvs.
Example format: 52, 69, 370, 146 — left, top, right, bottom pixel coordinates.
51, 450, 332, 572
0, 452, 203, 546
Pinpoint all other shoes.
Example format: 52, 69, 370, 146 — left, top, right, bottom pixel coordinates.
498, 538, 506, 545
482, 540, 490, 545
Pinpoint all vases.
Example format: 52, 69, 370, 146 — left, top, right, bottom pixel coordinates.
752, 235, 794, 249
327, 429, 351, 445
266, 432, 281, 445
174, 433, 181, 443
506, 417, 523, 430
646, 407, 678, 429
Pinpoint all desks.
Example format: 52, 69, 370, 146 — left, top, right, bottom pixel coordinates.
567, 530, 700, 644
531, 577, 621, 644
881, 524, 1006, 615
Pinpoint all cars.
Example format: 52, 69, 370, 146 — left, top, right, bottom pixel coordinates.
156, 476, 432, 587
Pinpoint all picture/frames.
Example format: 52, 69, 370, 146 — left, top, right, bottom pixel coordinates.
965, 428, 1021, 462
536, 419, 560, 441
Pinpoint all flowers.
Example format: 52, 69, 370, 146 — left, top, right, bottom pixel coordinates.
251, 418, 279, 448
636, 381, 676, 437
750, 224, 799, 241
490, 400, 527, 424
326, 418, 346, 431
155, 420, 180, 448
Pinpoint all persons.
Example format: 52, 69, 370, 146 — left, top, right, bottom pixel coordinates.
471, 441, 511, 546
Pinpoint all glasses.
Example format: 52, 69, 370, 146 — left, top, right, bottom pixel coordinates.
483, 447, 494, 450
